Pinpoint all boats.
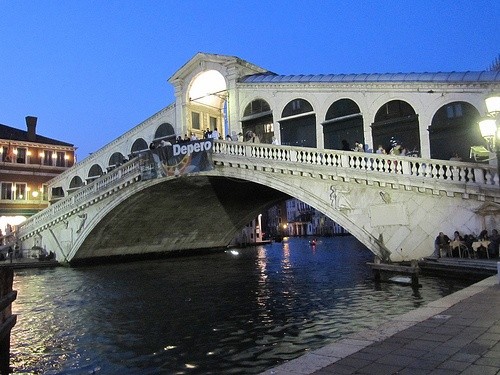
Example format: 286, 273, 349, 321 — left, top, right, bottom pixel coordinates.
310, 241, 318, 246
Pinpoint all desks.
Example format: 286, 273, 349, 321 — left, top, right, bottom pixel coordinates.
472, 241, 493, 260
448, 241, 464, 258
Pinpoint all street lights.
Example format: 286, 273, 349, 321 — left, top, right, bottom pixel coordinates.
477, 87, 500, 192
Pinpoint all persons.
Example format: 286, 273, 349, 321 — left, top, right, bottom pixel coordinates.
376, 137, 419, 171
351, 142, 364, 152
237, 133, 243, 142
14, 243, 21, 260
99, 154, 133, 177
226, 135, 232, 141
203, 127, 219, 140
45, 251, 53, 261
248, 133, 260, 143
176, 133, 197, 142
452, 229, 500, 261
435, 232, 450, 258
8, 246, 13, 263
150, 139, 172, 149
